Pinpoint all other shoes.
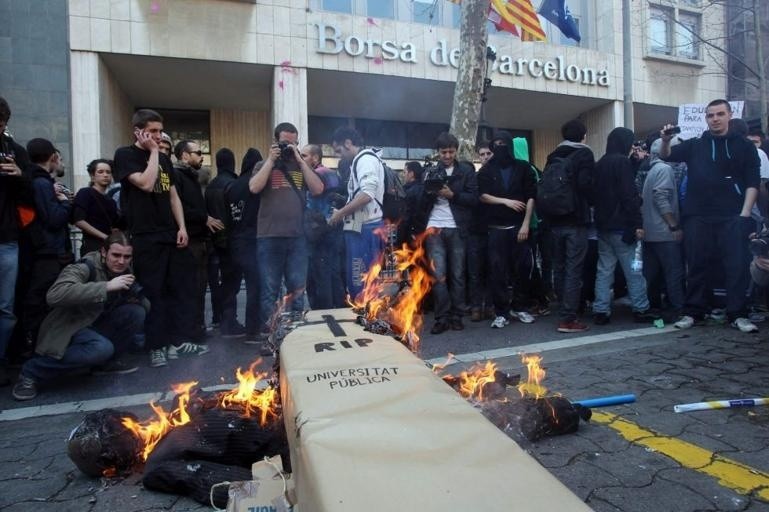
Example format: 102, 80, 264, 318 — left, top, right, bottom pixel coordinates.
592, 312, 613, 324
556, 320, 591, 332
486, 306, 497, 320
526, 306, 551, 316
451, 315, 464, 331
471, 308, 481, 322
653, 319, 665, 328
710, 309, 725, 319
433, 319, 449, 331
748, 312, 765, 322
634, 310, 663, 323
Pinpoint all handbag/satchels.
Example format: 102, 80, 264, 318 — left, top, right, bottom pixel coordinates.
304, 207, 329, 249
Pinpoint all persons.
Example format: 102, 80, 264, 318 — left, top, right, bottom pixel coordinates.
65, 371, 591, 507
0, 96, 769, 400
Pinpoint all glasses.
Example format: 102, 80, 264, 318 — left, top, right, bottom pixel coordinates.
190, 150, 202, 156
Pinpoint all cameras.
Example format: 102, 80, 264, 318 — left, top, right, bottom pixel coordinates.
277, 140, 294, 160
424, 161, 464, 191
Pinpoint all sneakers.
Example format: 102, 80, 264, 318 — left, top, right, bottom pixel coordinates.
731, 319, 758, 331
491, 315, 511, 329
674, 314, 706, 328
13, 374, 37, 400
508, 310, 535, 323
96, 321, 275, 376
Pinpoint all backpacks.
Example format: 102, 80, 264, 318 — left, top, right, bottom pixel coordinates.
354, 152, 407, 224
536, 156, 576, 221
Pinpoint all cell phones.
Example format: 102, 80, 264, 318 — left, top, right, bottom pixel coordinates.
664, 125, 682, 133
136, 129, 148, 138
121, 280, 146, 300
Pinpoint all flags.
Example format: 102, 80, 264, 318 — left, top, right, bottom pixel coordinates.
534, 0, 582, 43
485, 1, 545, 43
